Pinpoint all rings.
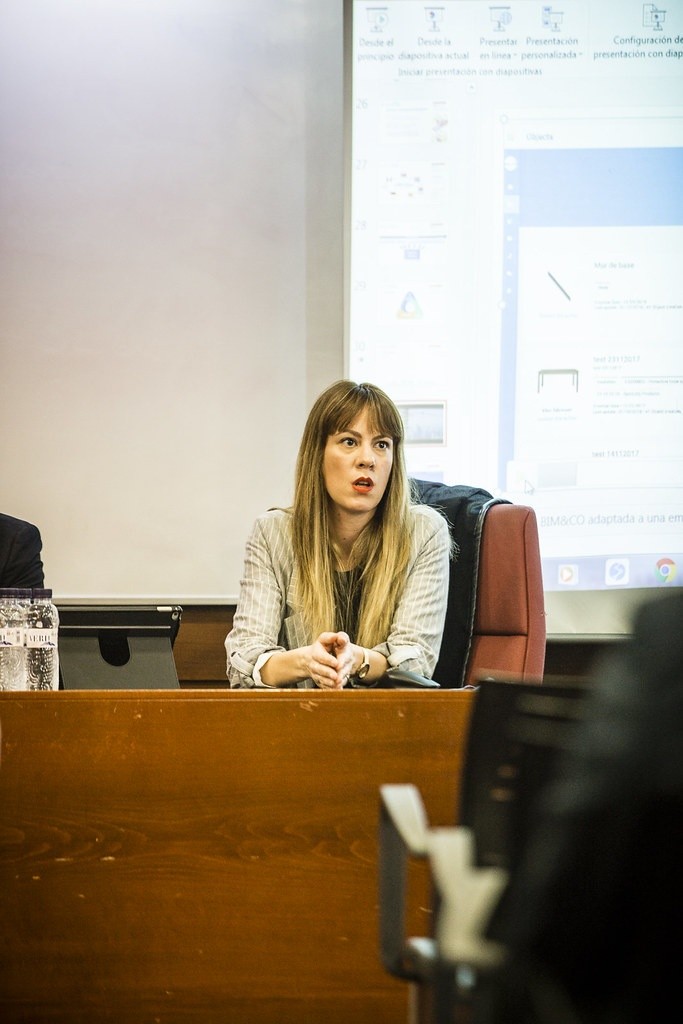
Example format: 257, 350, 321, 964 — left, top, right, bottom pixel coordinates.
346, 674, 350, 682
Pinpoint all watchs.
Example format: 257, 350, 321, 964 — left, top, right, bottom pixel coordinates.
350, 646, 370, 679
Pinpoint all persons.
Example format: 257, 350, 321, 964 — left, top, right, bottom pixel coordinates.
224, 379, 450, 690
0, 512, 64, 690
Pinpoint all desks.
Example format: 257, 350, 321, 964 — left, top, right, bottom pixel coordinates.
0, 688, 479, 1024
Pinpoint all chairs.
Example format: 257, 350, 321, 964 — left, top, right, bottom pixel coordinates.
379, 590, 683, 1024
408, 479, 546, 689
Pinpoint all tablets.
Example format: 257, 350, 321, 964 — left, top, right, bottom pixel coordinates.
54, 606, 183, 690
